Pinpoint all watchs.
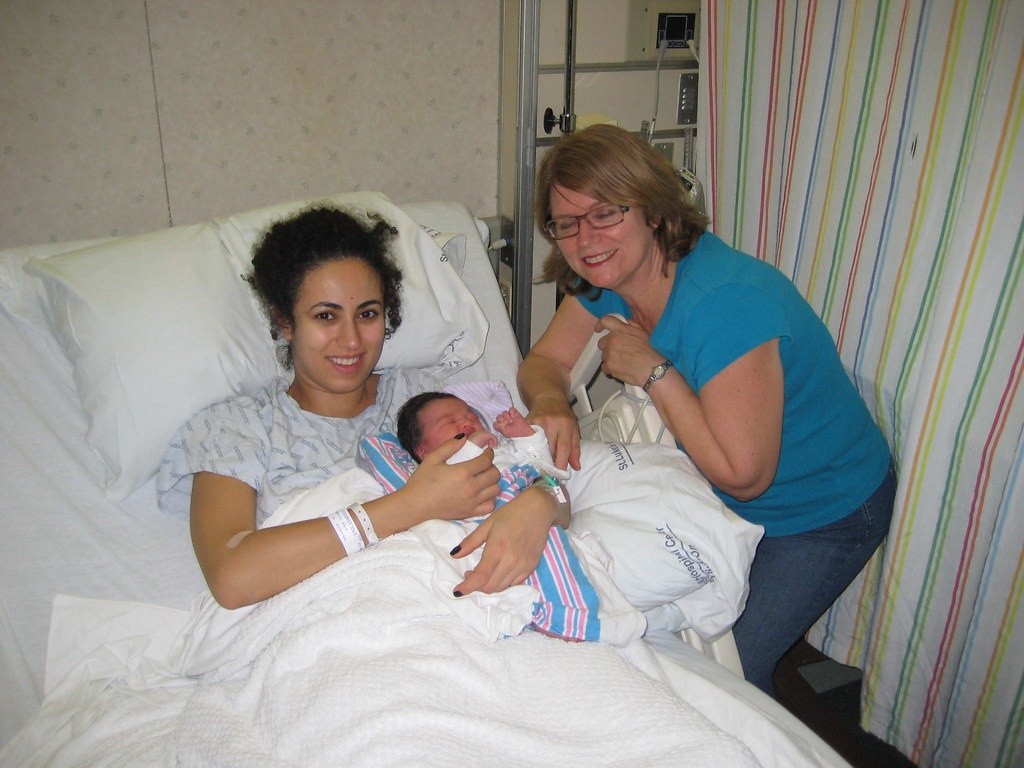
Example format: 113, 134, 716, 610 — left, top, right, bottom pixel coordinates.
643, 361, 673, 392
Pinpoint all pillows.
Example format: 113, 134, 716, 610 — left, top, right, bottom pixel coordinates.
566, 439, 767, 649
20, 190, 490, 506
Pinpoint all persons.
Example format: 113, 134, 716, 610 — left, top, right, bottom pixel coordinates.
157, 207, 762, 768
515, 125, 898, 697
397, 391, 647, 643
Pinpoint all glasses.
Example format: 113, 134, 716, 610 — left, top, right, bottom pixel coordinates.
542, 204, 630, 240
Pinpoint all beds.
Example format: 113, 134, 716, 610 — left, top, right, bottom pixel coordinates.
0, 200, 853, 768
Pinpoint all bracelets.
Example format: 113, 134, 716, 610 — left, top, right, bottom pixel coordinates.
328, 502, 378, 556
